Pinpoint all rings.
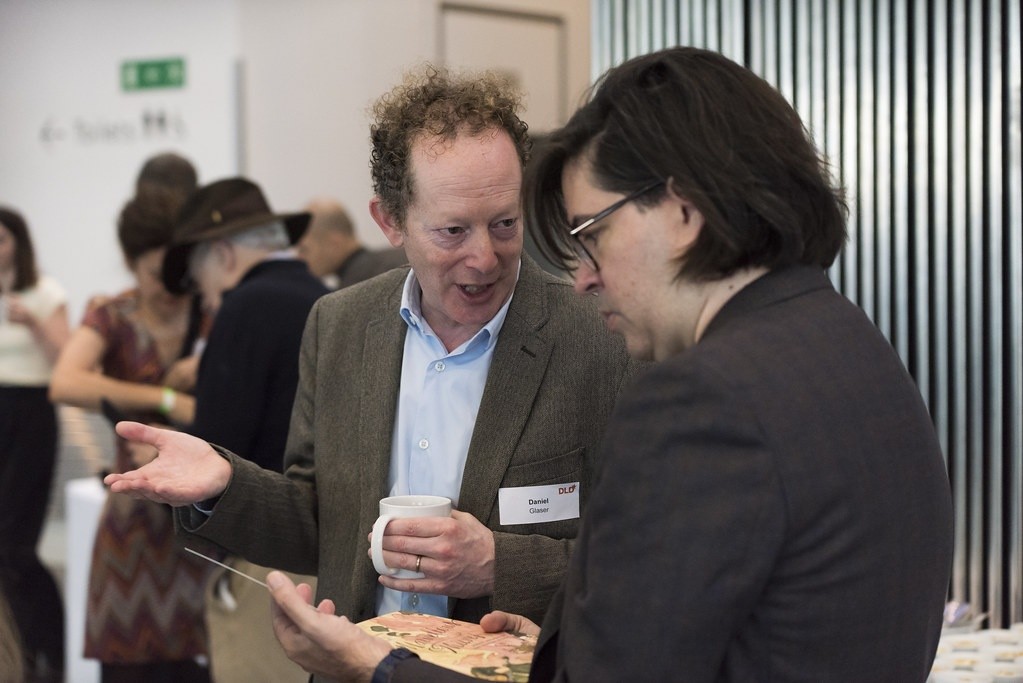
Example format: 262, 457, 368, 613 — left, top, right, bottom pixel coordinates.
416, 555, 422, 572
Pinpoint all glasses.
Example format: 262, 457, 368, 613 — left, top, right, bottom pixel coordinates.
571, 175, 664, 272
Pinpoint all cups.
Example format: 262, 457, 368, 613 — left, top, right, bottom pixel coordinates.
369, 494, 454, 581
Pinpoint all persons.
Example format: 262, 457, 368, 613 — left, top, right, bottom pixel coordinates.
109, 63, 644, 681
1, 152, 372, 679
267, 42, 955, 681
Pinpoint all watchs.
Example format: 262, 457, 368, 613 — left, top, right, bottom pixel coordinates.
369, 645, 420, 683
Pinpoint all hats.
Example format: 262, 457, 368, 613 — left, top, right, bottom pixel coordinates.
160, 177, 314, 296
